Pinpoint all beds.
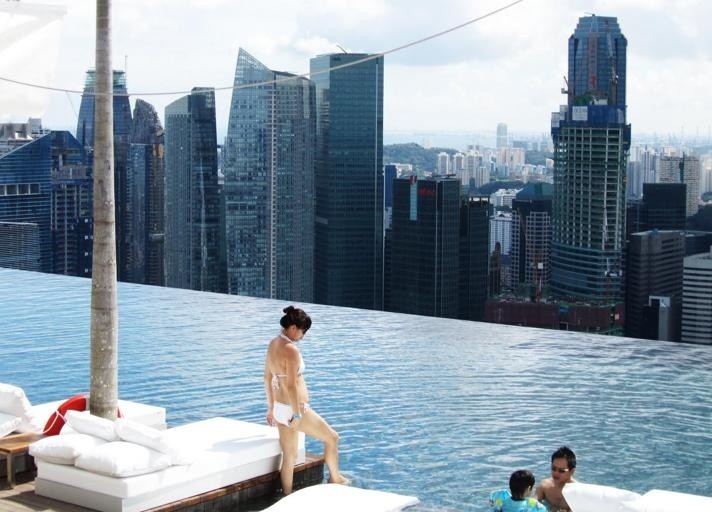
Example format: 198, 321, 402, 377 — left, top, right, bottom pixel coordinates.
30, 418, 308, 510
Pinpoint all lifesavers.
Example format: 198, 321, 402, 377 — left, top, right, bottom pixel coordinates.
43, 394, 121, 436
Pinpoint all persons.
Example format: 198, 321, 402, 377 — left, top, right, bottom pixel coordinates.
535, 446, 576, 512
264, 306, 352, 495
490, 470, 548, 512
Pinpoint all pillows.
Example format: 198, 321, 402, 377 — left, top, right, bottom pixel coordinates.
0, 381, 187, 479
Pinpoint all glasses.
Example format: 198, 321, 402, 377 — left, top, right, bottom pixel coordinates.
552, 466, 574, 473
303, 327, 309, 334
